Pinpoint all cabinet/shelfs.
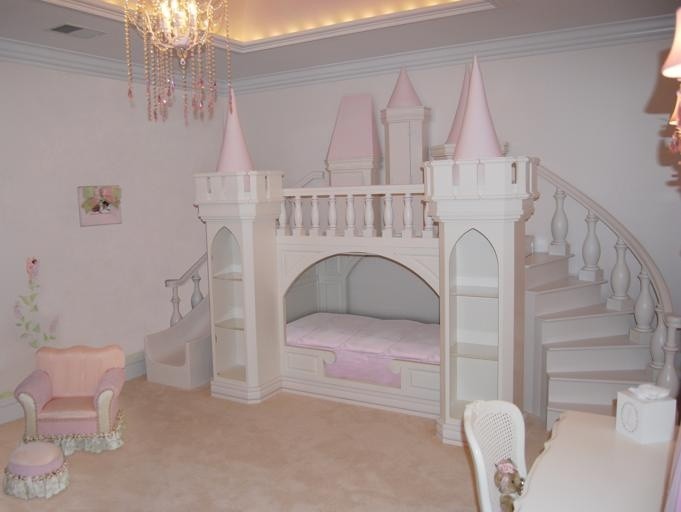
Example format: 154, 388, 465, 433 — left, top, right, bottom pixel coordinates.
203, 216, 279, 403
442, 218, 517, 445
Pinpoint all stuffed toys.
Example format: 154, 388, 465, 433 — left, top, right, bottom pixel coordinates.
495, 457, 524, 512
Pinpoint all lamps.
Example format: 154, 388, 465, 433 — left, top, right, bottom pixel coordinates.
663, 7, 681, 179
121, 0, 231, 121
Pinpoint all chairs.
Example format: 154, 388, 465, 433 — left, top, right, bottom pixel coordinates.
463, 395, 529, 510
13, 342, 127, 454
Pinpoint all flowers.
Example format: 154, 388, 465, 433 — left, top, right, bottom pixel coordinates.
13, 255, 63, 349
79, 184, 123, 216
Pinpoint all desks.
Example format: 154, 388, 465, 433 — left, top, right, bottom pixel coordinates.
519, 407, 677, 510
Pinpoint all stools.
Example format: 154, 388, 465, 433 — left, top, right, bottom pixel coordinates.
4, 440, 70, 498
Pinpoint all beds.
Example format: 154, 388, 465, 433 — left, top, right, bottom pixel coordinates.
282, 310, 441, 415
